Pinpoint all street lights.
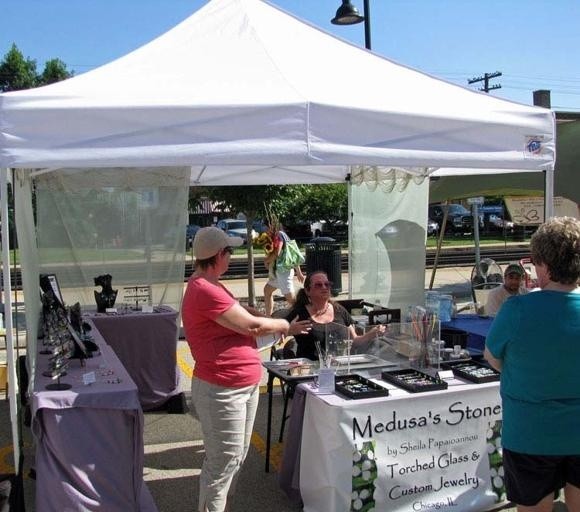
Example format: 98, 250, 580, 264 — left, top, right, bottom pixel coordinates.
331, 0, 371, 50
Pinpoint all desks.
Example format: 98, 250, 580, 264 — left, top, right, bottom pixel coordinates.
262, 299, 560, 512
30, 305, 180, 512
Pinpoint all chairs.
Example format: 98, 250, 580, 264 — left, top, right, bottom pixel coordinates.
519, 258, 540, 288
0, 334, 8, 402
471, 257, 505, 315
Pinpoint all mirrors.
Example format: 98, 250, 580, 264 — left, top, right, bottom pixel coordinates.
47, 273, 64, 306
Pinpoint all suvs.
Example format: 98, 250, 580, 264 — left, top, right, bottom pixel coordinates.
429, 204, 474, 235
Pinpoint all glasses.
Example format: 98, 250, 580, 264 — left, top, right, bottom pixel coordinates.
311, 283, 330, 288
222, 247, 234, 255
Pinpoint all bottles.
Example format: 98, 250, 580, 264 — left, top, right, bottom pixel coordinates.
405, 305, 414, 336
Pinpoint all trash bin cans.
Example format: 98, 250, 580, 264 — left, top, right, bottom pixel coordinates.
304, 237, 345, 297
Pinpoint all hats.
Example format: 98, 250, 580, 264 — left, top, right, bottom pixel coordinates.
504, 264, 523, 276
192, 227, 243, 260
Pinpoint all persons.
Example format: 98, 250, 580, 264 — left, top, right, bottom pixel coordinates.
484, 264, 536, 319
281, 269, 388, 360
179, 225, 291, 512
481, 214, 580, 512
260, 214, 304, 317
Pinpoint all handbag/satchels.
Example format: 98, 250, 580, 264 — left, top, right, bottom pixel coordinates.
276, 239, 305, 269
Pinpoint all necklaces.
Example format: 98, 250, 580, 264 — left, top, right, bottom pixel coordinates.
308, 303, 330, 316
101, 290, 116, 305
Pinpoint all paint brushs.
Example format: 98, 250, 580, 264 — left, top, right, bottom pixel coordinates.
411, 311, 436, 375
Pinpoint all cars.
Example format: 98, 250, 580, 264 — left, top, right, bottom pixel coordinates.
164, 219, 261, 251
291, 204, 440, 247
477, 215, 514, 236
